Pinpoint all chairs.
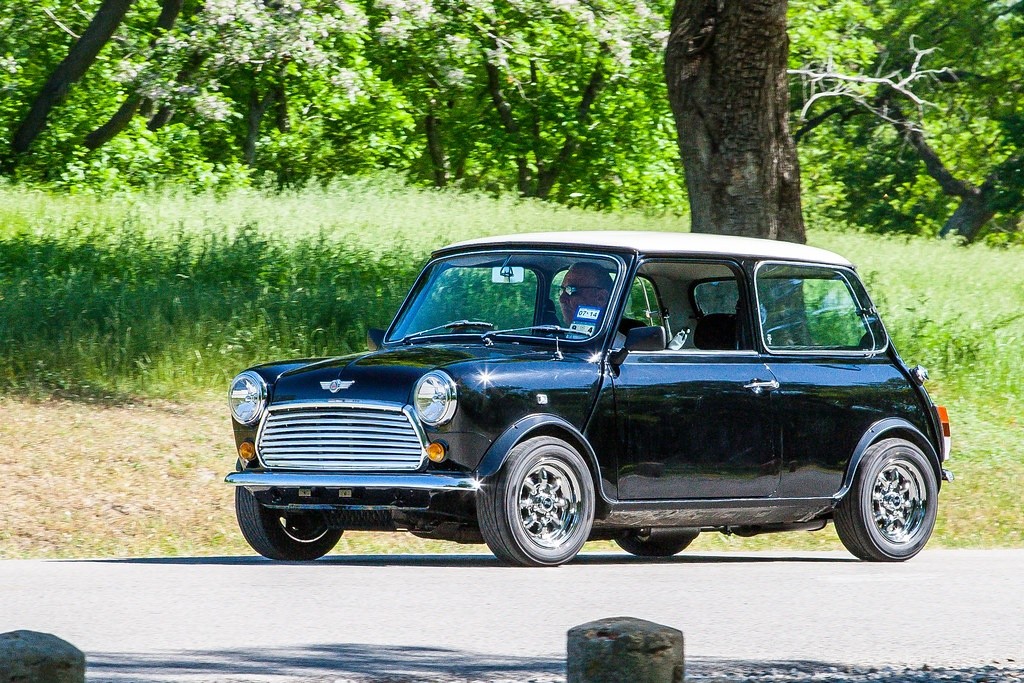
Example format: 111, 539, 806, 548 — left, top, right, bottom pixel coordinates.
693, 312, 737, 349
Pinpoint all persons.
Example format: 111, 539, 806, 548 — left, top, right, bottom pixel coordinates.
559, 262, 648, 351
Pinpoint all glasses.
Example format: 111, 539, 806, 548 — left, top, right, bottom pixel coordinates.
556, 284, 602, 296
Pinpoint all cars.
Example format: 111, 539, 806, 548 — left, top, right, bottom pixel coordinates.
224, 230, 954, 567
